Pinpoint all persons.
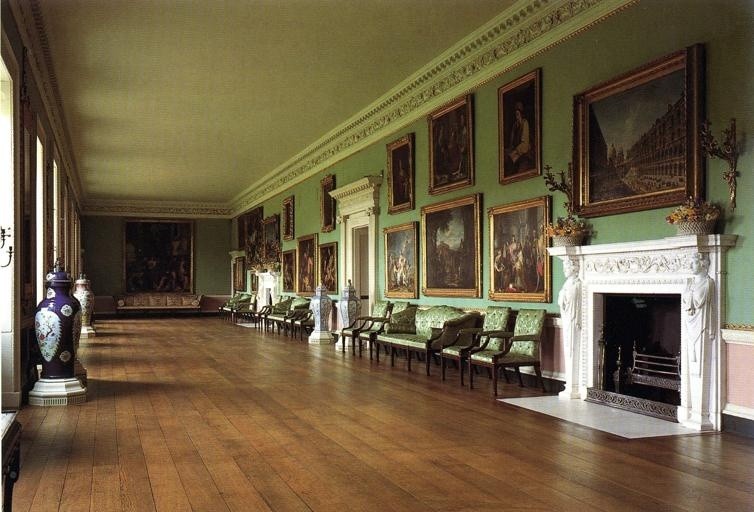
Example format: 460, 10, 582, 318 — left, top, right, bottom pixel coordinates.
679, 249, 717, 423
493, 225, 543, 294
503, 102, 530, 169
298, 241, 335, 290
123, 235, 188, 292
393, 156, 409, 202
557, 260, 583, 400
384, 249, 414, 294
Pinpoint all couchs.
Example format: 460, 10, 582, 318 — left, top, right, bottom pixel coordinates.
113, 292, 205, 318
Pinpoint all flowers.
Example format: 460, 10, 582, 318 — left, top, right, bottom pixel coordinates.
544, 216, 588, 237
664, 196, 722, 225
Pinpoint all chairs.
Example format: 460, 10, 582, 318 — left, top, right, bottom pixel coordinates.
216, 294, 313, 341
342, 301, 547, 396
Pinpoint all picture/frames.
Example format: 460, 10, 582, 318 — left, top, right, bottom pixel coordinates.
572, 43, 706, 218
122, 220, 194, 294
236, 174, 338, 297
383, 68, 552, 303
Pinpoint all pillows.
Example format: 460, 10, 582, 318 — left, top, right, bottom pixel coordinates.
118, 295, 199, 306
